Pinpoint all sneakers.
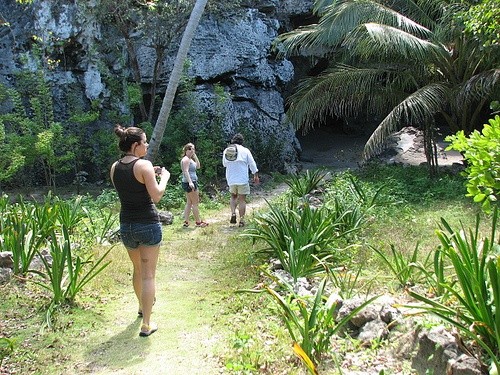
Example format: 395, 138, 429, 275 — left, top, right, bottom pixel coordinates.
196, 222, 209, 227
239, 222, 244, 227
139, 322, 157, 336
183, 221, 190, 226
137, 296, 156, 315
230, 213, 237, 223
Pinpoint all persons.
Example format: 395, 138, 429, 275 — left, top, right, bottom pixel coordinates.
181, 143, 209, 228
110, 125, 170, 336
223, 134, 259, 227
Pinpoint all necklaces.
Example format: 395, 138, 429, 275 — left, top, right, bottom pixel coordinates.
126, 154, 137, 157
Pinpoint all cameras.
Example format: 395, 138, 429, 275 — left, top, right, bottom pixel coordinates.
155, 168, 162, 174
